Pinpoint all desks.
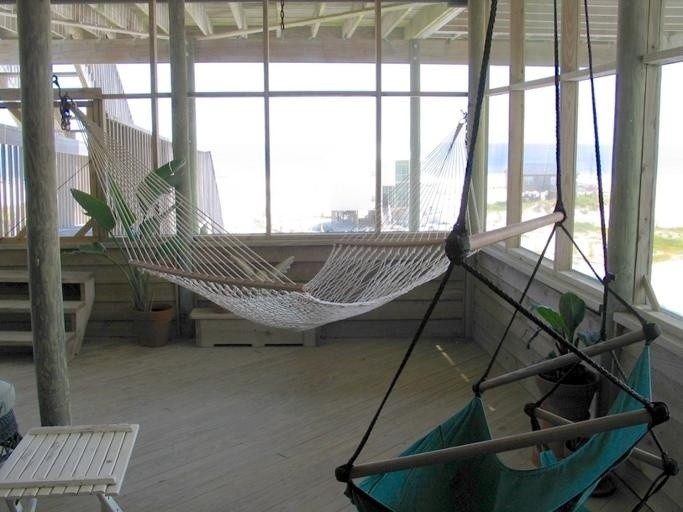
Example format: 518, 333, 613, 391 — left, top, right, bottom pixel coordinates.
1, 421, 141, 511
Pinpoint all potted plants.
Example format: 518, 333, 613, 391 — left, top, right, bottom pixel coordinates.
55, 158, 191, 348
525, 291, 605, 426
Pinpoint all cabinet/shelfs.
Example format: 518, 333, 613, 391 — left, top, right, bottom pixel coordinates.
189, 307, 319, 346
